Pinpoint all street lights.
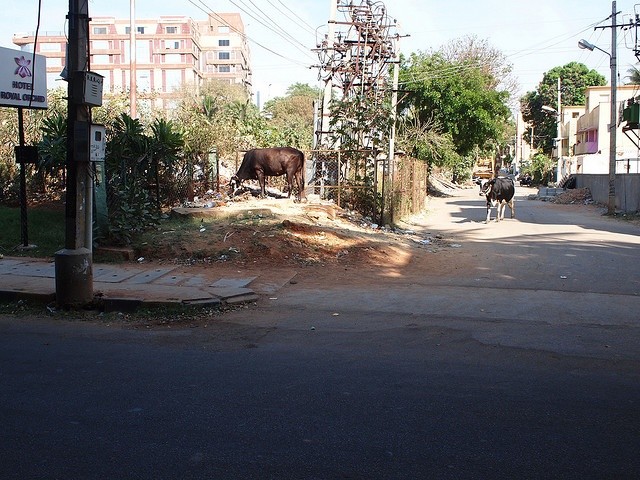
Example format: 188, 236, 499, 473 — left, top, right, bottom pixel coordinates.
541, 103, 562, 181
579, 37, 616, 216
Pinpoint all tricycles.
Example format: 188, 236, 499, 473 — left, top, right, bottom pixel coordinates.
519, 173, 532, 187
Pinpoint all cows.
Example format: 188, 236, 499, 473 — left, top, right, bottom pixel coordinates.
228, 146, 305, 202
475, 176, 515, 223
